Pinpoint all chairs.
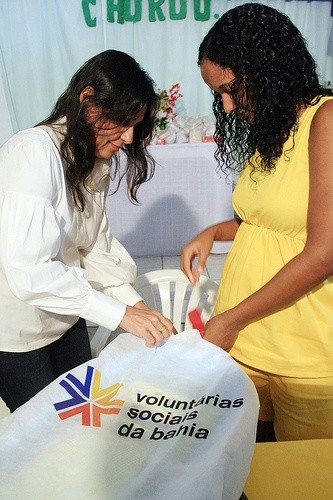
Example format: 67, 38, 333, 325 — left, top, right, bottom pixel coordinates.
90, 269, 220, 358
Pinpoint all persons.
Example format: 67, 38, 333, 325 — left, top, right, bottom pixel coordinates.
1, 48, 181, 413
178, 1, 333, 444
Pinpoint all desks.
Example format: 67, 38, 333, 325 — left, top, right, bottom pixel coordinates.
240, 438, 333, 500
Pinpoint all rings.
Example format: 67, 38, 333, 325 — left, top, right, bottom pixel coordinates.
160, 325, 167, 334
